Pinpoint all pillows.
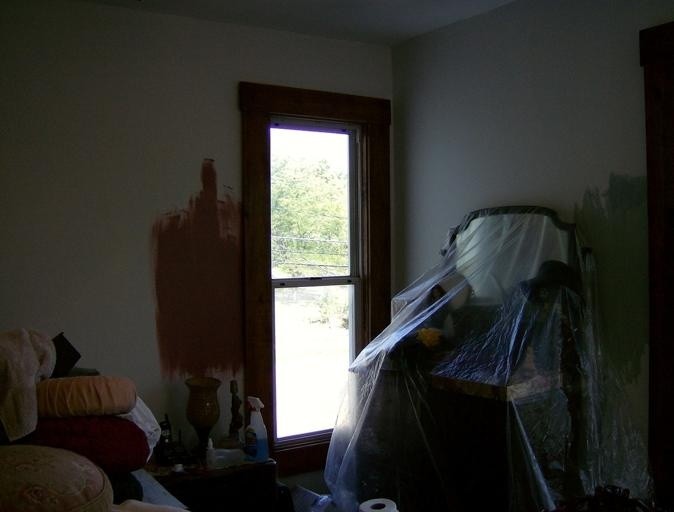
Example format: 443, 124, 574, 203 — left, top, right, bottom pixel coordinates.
0, 373, 151, 511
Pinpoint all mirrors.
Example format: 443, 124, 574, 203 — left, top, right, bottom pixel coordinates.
446, 204, 583, 310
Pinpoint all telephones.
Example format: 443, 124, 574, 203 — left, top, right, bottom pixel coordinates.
155, 413, 174, 467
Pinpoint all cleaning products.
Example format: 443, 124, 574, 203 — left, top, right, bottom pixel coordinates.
244, 396, 270, 464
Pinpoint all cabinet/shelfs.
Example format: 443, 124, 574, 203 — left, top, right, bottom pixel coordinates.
349, 357, 588, 511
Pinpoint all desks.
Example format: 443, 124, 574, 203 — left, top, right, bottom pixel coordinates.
152, 445, 281, 512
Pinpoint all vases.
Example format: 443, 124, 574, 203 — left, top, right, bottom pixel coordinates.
182, 376, 224, 465
